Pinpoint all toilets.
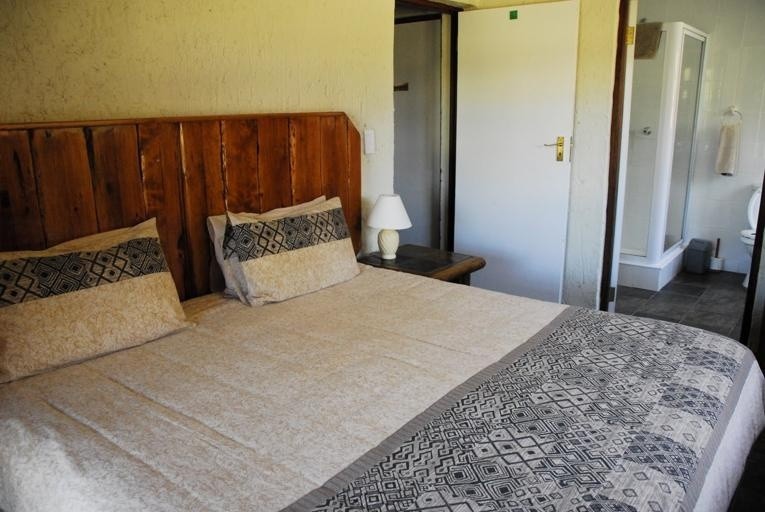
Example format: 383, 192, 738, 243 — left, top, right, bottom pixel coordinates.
739, 183, 762, 288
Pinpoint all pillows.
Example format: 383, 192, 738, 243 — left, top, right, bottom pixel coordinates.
0, 215, 197, 385
206, 192, 363, 308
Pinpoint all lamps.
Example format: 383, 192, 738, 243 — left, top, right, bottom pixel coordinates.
367, 193, 413, 261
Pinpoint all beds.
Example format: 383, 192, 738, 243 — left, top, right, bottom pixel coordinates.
0, 113, 764, 512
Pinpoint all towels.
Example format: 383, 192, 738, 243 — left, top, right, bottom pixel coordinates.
713, 123, 741, 177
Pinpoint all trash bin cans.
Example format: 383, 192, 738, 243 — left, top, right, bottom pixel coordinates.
686, 239, 712, 275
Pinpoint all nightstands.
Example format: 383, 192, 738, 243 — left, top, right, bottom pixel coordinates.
357, 243, 486, 286
